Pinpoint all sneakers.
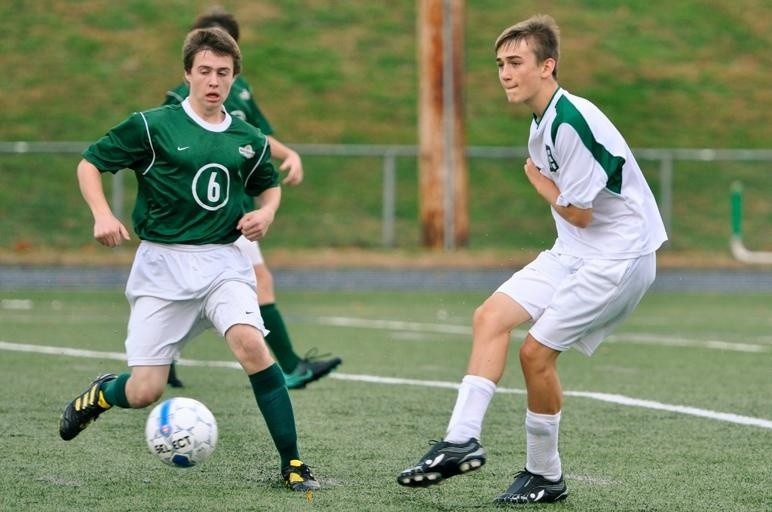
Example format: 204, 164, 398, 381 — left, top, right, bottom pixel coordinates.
493, 468, 569, 504
397, 437, 486, 487
167, 376, 182, 388
60, 373, 118, 441
283, 359, 341, 389
282, 459, 320, 491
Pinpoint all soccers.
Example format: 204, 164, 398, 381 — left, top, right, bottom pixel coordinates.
146, 397, 218, 467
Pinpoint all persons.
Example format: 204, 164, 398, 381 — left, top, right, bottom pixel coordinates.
159, 7, 345, 392
56, 23, 322, 495
395, 12, 670, 505
